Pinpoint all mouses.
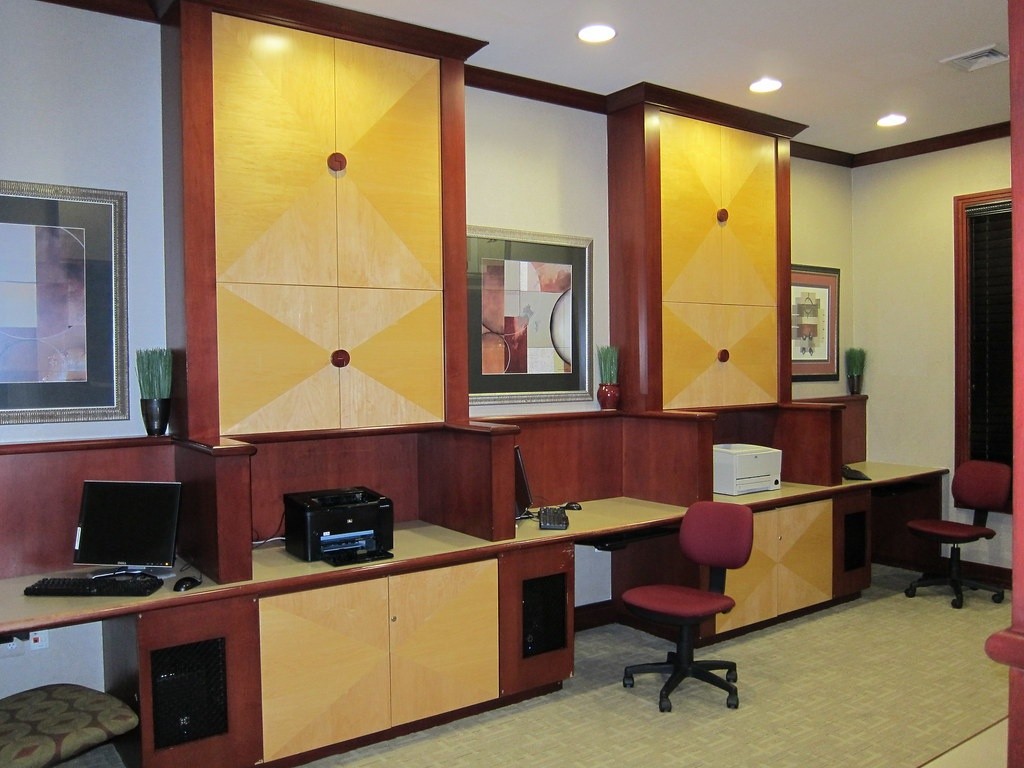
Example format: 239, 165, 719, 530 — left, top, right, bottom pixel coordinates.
842, 466, 851, 470
564, 502, 581, 510
173, 576, 202, 592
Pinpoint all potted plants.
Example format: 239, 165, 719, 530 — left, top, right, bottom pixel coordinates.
136, 347, 172, 436
844, 349, 866, 394
595, 344, 620, 412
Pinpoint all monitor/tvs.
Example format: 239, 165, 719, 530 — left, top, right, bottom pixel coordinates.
73, 481, 182, 580
514, 444, 533, 519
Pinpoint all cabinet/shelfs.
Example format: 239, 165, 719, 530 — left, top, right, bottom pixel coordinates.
159, 0, 488, 442
607, 81, 808, 410
248, 555, 504, 768
715, 499, 833, 641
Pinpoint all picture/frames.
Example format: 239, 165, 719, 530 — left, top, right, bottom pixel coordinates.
465, 225, 597, 407
0, 176, 131, 428
790, 264, 840, 382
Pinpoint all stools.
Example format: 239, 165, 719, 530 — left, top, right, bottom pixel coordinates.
0, 684, 138, 768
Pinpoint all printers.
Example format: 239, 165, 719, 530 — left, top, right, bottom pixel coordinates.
284, 486, 395, 567
713, 443, 783, 495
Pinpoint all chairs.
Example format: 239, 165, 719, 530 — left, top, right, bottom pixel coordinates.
619, 500, 754, 711
904, 460, 1011, 608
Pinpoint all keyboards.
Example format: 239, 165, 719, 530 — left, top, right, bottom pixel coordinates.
841, 469, 872, 481
538, 507, 567, 530
23, 577, 164, 597
